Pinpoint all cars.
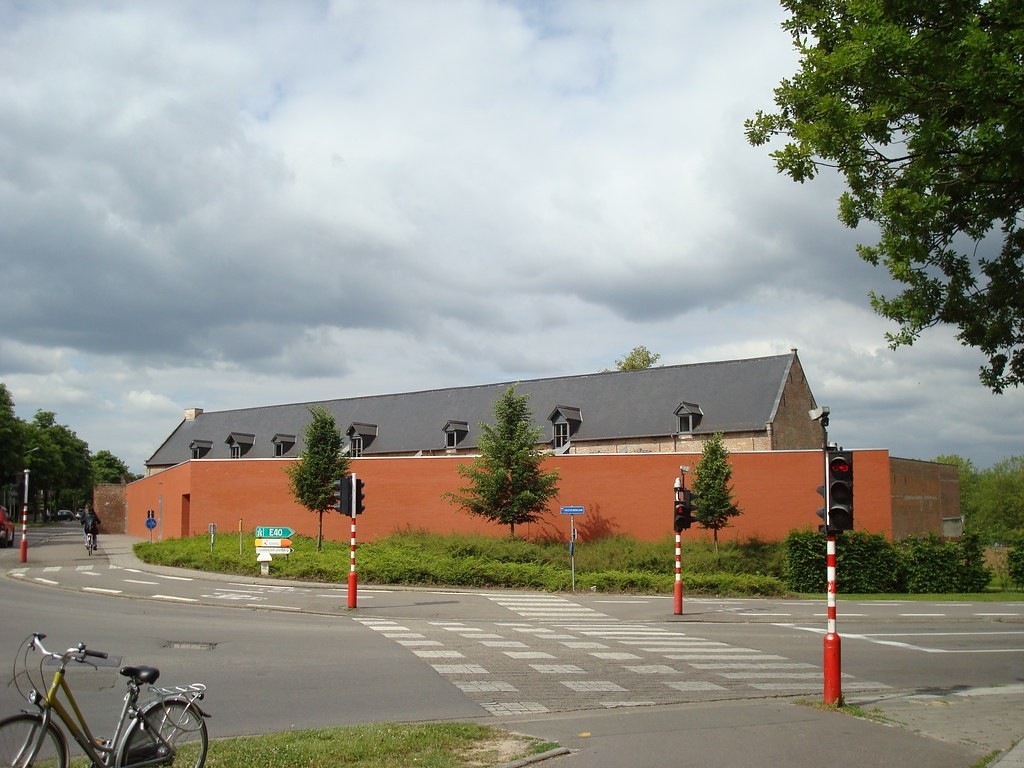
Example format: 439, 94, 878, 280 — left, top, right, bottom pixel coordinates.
48, 509, 84, 523
0, 505, 14, 548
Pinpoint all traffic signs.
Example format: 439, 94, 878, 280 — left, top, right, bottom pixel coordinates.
256, 527, 296, 539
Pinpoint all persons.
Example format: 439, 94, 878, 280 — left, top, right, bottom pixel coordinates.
79, 501, 99, 550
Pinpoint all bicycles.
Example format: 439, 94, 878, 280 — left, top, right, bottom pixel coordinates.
0, 632, 212, 768
84, 533, 93, 556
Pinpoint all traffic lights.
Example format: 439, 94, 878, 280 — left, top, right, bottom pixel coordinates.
356, 479, 365, 514
332, 478, 352, 516
684, 490, 699, 528
816, 485, 826, 533
829, 451, 854, 531
674, 501, 686, 528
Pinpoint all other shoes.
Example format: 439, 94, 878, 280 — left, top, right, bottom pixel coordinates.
93, 545, 97, 550
